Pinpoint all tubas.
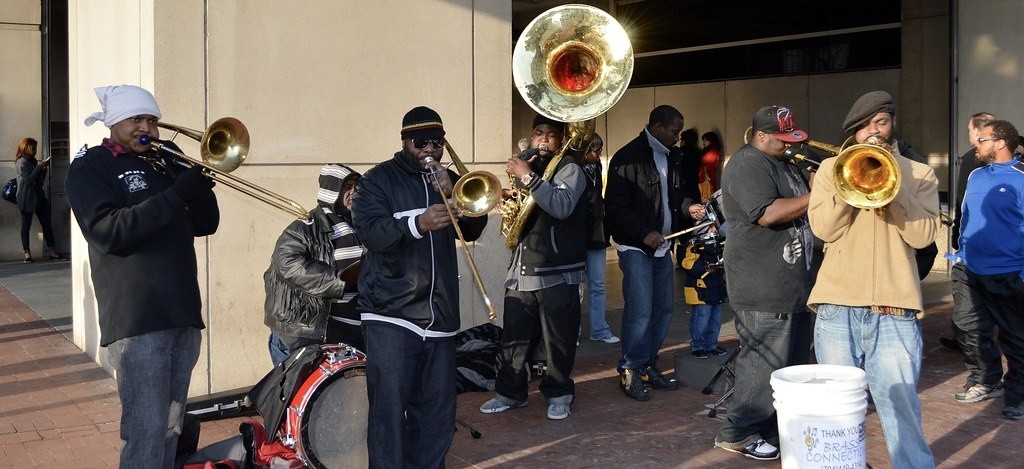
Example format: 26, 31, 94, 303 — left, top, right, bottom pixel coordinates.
833, 136, 903, 220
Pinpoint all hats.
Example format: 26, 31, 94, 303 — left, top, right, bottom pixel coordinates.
753, 105, 808, 143
681, 128, 698, 144
532, 114, 564, 132
400, 106, 446, 140
842, 91, 895, 133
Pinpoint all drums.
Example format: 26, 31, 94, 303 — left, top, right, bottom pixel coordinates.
278, 341, 370, 469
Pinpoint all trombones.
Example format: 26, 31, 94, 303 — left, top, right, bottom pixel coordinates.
744, 124, 859, 176
151, 118, 313, 225
428, 138, 501, 322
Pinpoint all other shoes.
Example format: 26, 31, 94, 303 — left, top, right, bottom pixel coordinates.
49, 255, 69, 262
24, 257, 33, 264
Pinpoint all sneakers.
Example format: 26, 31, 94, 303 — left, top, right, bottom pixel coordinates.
715, 437, 781, 461
1001, 402, 1024, 420
692, 350, 708, 359
619, 368, 650, 401
479, 398, 529, 414
641, 367, 679, 391
954, 380, 1004, 404
705, 346, 728, 356
546, 403, 569, 420
599, 334, 620, 344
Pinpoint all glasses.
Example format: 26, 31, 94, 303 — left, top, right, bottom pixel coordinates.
410, 139, 447, 151
1015, 149, 1024, 159
977, 138, 999, 144
680, 138, 684, 142
591, 149, 602, 155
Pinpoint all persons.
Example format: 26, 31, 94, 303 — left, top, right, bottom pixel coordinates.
943, 119, 1024, 421
64, 85, 220, 469
681, 212, 730, 360
676, 128, 703, 269
262, 161, 369, 369
938, 111, 1024, 356
14, 138, 70, 264
862, 124, 941, 418
604, 104, 707, 403
713, 105, 822, 463
350, 106, 489, 468
1012, 135, 1024, 162
574, 131, 621, 348
479, 112, 593, 420
806, 91, 941, 469
698, 130, 722, 207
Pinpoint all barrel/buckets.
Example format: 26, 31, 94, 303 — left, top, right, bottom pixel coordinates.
769, 363, 867, 469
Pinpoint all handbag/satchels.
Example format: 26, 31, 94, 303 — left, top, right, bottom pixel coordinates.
698, 166, 714, 205
2, 174, 21, 204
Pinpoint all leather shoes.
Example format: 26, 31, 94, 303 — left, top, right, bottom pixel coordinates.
940, 337, 961, 352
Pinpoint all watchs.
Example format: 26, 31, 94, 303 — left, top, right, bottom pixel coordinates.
520, 172, 536, 187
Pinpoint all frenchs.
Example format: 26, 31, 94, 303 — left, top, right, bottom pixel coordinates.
502, 3, 634, 248
